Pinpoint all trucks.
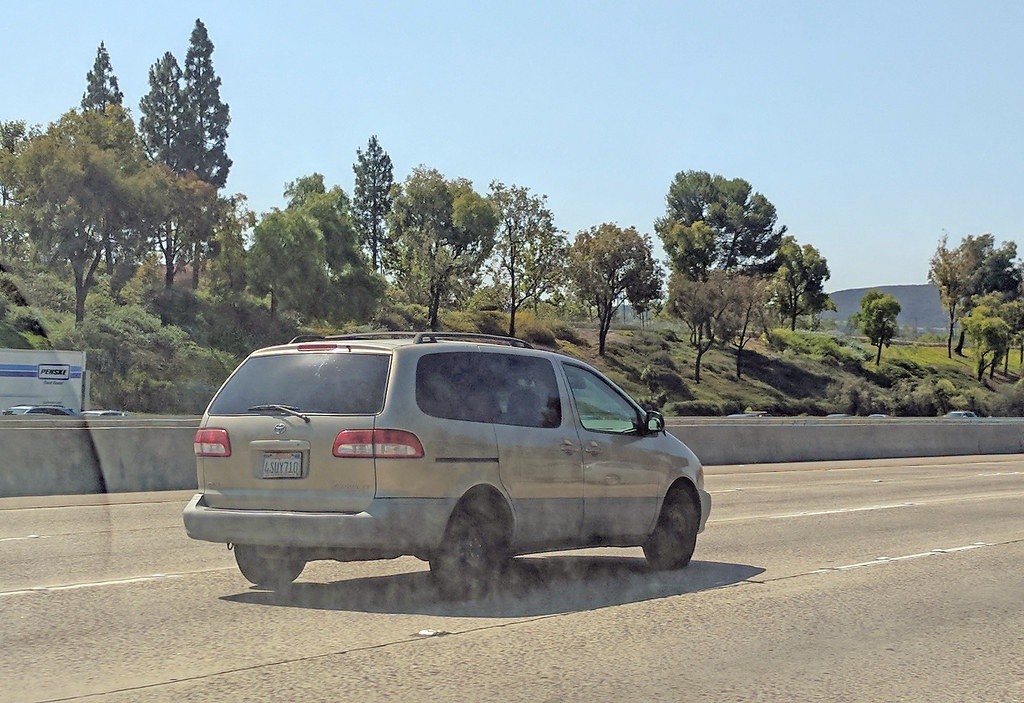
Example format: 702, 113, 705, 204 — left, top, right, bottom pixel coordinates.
0, 347, 85, 415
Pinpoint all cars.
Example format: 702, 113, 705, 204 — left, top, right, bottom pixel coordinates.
943, 411, 977, 418
2, 405, 79, 415
78, 411, 128, 417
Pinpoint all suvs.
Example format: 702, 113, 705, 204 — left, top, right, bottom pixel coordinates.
181, 332, 710, 604
